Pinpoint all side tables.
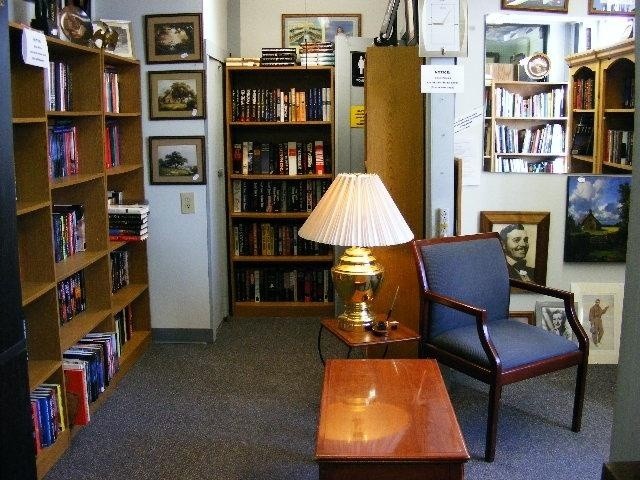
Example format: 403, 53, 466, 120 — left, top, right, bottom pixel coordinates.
318, 314, 421, 367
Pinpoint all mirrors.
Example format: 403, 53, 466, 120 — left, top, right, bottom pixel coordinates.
484, 13, 636, 174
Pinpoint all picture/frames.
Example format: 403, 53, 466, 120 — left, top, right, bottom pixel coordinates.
481, 211, 626, 365
149, 136, 206, 185
145, 13, 203, 65
281, 13, 360, 66
148, 70, 207, 120
500, 0, 569, 13
588, 0, 635, 15
104, 20, 136, 59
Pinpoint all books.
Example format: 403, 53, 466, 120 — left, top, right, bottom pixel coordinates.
23, 317, 31, 363
572, 70, 635, 169
58, 269, 89, 327
46, 62, 73, 112
61, 330, 120, 427
109, 250, 131, 296
107, 189, 149, 241
105, 121, 120, 169
48, 118, 79, 179
114, 303, 134, 349
227, 40, 337, 305
483, 82, 567, 175
104, 63, 120, 114
52, 204, 87, 262
32, 383, 66, 450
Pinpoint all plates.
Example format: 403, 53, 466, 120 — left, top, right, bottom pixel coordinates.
91, 20, 113, 50
60, 5, 94, 43
523, 52, 551, 78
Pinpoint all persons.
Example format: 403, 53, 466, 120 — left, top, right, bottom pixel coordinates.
357, 55, 365, 76
549, 308, 571, 340
588, 298, 610, 349
498, 224, 536, 284
335, 26, 345, 38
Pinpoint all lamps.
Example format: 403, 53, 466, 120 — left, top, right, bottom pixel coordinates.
298, 173, 415, 332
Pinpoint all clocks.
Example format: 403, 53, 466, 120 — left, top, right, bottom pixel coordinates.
418, 0, 468, 58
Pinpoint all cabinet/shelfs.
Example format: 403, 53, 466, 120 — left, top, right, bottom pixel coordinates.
485, 80, 569, 174
365, 46, 425, 333
564, 37, 635, 175
8, 20, 152, 480
226, 65, 334, 318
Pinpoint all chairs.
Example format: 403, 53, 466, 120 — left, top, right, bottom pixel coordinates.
414, 231, 589, 463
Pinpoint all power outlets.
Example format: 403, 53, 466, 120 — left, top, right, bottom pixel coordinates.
180, 192, 194, 214
